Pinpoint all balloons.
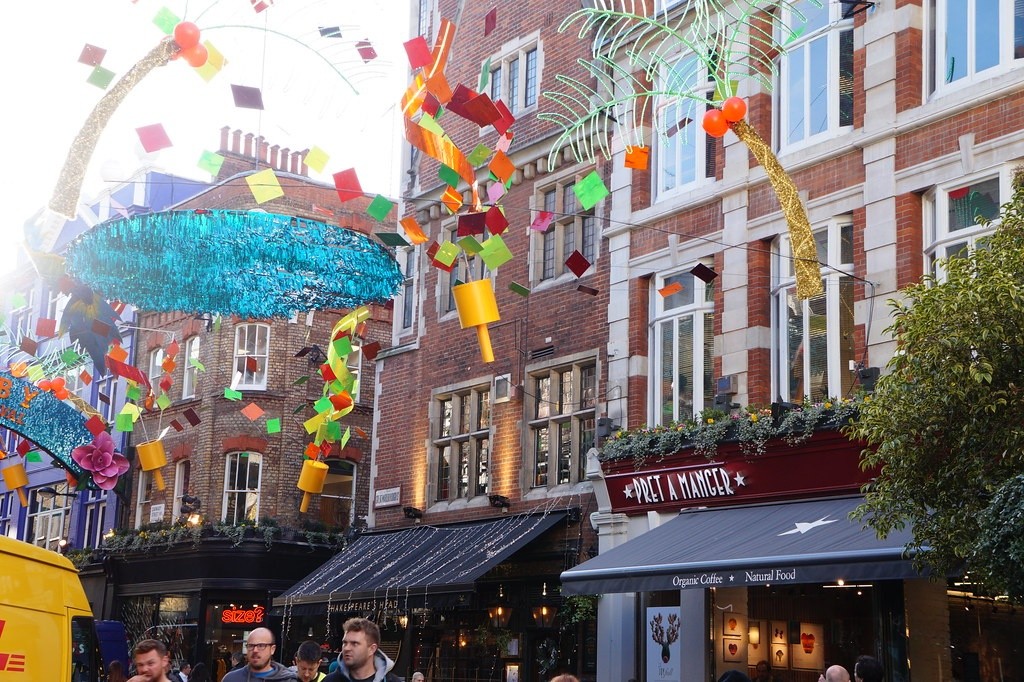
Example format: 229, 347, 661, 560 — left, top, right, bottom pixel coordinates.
702, 97, 746, 138
160, 21, 208, 68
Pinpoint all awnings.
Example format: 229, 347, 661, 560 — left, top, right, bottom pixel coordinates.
272, 506, 577, 605
560, 493, 930, 596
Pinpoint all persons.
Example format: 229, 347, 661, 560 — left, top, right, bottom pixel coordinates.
817, 654, 884, 682
412, 672, 424, 682
551, 675, 578, 682
718, 660, 775, 682
108, 618, 401, 682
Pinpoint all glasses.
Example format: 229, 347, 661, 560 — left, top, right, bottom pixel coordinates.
245, 643, 274, 651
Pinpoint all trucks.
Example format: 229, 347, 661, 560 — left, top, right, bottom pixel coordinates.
0, 535, 109, 682
